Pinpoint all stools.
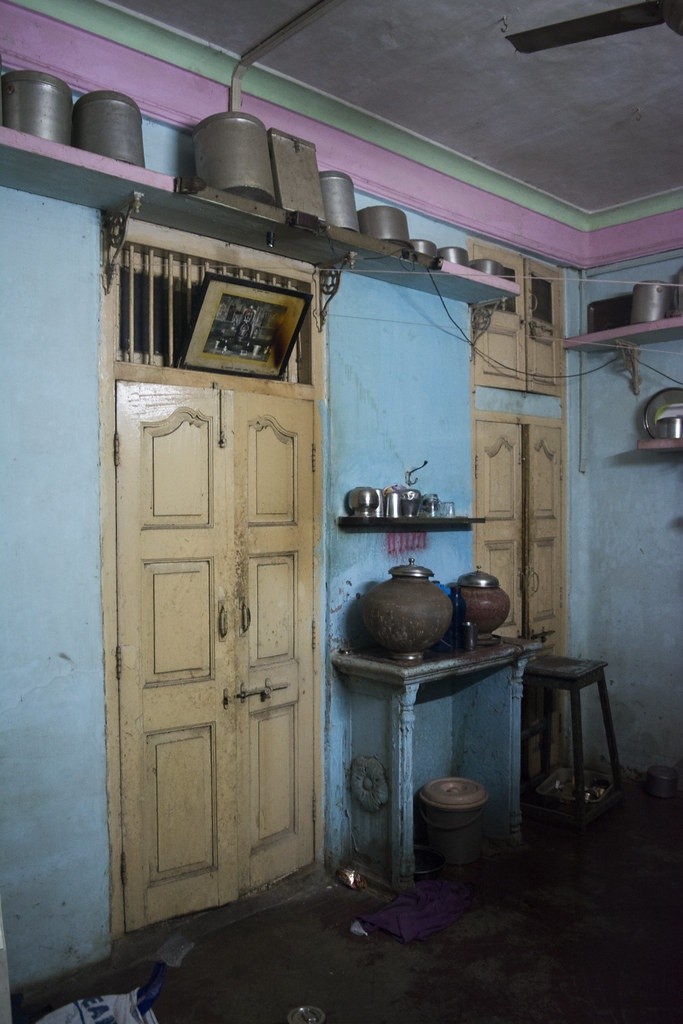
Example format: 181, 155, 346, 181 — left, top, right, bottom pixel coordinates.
522, 655, 623, 830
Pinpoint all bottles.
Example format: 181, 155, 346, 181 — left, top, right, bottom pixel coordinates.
431, 581, 466, 651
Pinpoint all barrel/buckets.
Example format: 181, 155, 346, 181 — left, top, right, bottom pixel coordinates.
469, 259, 507, 280
190, 112, 275, 206
71, 90, 145, 169
438, 247, 469, 267
1, 70, 73, 148
357, 206, 409, 246
410, 240, 437, 258
631, 280, 672, 324
319, 171, 360, 233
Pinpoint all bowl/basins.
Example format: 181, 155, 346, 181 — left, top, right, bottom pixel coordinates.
655, 417, 683, 439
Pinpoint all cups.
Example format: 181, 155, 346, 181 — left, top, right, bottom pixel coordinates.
386, 493, 401, 518
417, 494, 441, 518
462, 622, 478, 650
374, 488, 384, 518
441, 501, 455, 518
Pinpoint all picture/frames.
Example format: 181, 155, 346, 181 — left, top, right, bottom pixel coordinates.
177, 271, 313, 380
